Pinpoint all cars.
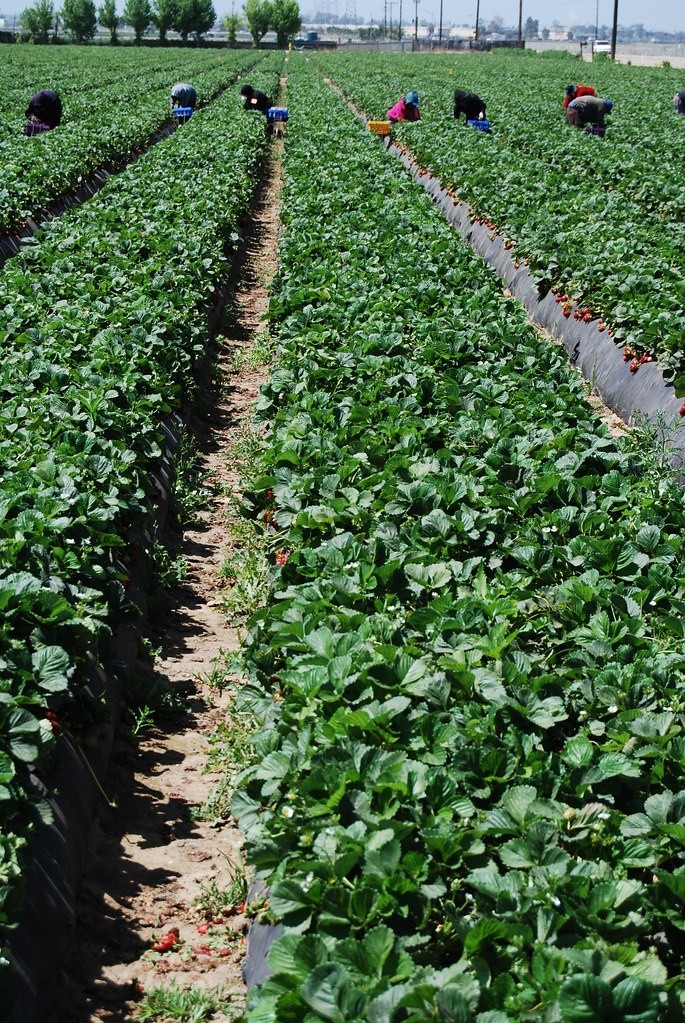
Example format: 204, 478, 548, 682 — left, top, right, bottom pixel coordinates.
593, 40, 611, 55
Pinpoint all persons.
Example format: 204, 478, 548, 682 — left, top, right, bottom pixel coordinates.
385, 90, 421, 123
673, 89, 685, 115
240, 84, 274, 124
24, 90, 62, 129
170, 83, 197, 125
453, 90, 492, 133
560, 83, 613, 131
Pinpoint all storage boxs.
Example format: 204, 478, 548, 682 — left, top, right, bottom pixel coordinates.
268, 106, 288, 122
367, 120, 391, 134
584, 128, 605, 137
468, 119, 489, 132
172, 107, 192, 116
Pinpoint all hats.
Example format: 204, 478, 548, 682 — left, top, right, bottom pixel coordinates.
406, 91, 420, 106
606, 100, 613, 115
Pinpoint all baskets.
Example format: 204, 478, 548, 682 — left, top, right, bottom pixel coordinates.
590, 122, 606, 137
172, 107, 192, 117
269, 107, 288, 118
26, 123, 50, 136
368, 121, 390, 134
468, 118, 488, 131
